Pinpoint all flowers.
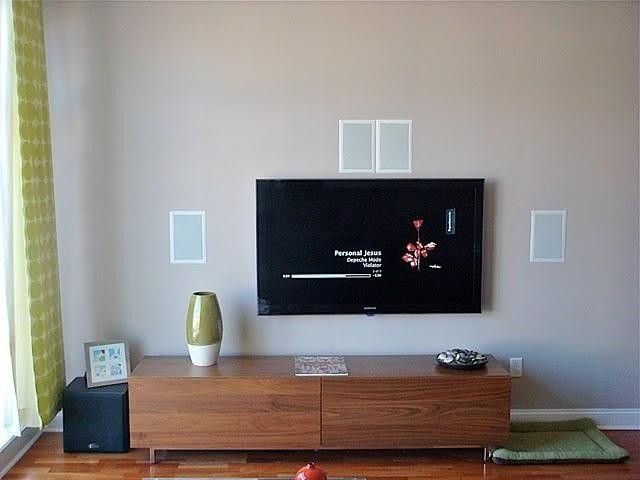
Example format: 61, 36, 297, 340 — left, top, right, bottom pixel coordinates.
402, 220, 437, 271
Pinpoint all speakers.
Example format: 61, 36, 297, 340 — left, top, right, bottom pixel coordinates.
62, 376, 129, 454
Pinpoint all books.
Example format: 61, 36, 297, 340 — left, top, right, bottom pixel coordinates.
293, 356, 348, 376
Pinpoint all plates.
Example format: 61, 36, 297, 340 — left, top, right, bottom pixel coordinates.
436, 353, 488, 371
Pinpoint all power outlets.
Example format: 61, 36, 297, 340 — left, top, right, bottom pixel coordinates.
509, 357, 524, 378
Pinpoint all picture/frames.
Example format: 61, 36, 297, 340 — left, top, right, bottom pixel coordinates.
83, 338, 131, 389
529, 209, 567, 263
375, 119, 413, 174
169, 210, 206, 265
338, 118, 375, 174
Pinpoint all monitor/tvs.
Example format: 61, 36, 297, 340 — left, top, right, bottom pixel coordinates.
256, 178, 485, 315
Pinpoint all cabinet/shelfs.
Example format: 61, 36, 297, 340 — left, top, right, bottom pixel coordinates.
127, 355, 512, 464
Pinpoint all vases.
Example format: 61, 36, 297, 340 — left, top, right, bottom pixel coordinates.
184, 291, 223, 368
